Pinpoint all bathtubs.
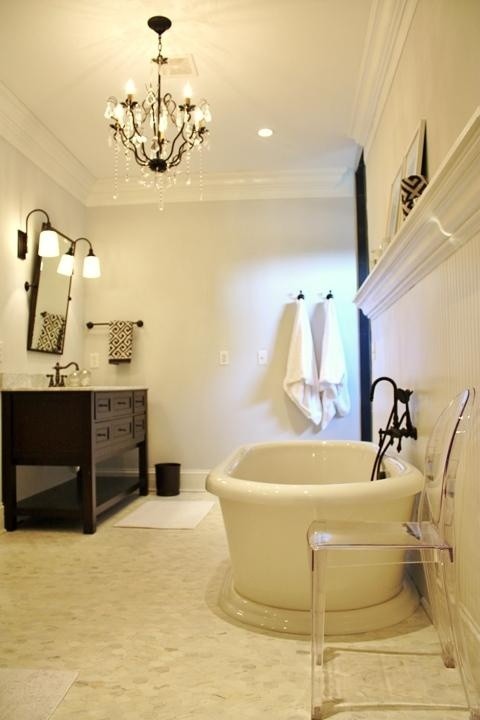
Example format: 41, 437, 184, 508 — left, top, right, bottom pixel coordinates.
207, 444, 424, 612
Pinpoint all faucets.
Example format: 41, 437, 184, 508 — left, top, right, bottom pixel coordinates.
55, 363, 79, 387
369, 376, 399, 434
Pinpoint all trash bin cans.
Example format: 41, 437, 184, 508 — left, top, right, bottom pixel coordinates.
155, 463, 180, 496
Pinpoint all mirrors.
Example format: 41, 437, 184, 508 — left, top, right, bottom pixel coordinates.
27, 221, 76, 355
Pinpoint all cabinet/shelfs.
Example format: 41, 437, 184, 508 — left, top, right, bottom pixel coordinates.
94, 389, 148, 445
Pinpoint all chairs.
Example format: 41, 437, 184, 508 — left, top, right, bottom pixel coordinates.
307, 384, 479, 719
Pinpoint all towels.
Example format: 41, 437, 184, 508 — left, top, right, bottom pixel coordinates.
37, 314, 66, 352
109, 321, 133, 365
282, 298, 351, 432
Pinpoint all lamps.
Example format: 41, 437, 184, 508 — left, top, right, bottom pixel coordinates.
17, 208, 60, 260
105, 16, 211, 174
75, 238, 101, 278
57, 245, 74, 276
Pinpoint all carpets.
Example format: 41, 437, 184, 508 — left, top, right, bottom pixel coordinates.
113, 500, 214, 530
0, 669, 79, 720
